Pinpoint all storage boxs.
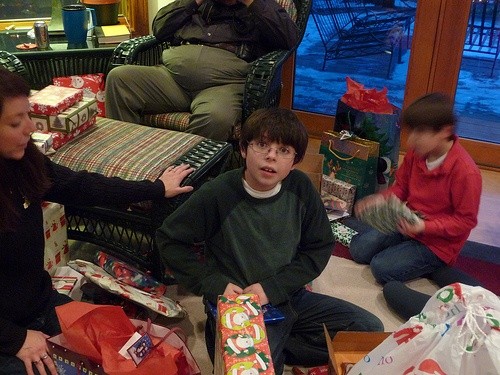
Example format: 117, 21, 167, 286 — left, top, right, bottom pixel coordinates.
320, 174, 356, 215
292, 153, 324, 194
28, 90, 98, 133
330, 220, 359, 248
213, 293, 276, 375
91, 250, 167, 297
53, 72, 106, 117
34, 115, 96, 150
323, 322, 393, 375
28, 84, 83, 116
53, 264, 87, 302
39, 201, 69, 277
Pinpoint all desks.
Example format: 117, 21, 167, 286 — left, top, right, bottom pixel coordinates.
49, 40, 96, 50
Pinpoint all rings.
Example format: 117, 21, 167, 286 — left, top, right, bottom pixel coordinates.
34, 359, 41, 365
41, 354, 48, 359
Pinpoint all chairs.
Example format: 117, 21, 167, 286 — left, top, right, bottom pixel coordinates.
463, 0, 500, 79
111, 0, 418, 152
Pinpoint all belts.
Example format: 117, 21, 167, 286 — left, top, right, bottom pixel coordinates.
171, 37, 251, 62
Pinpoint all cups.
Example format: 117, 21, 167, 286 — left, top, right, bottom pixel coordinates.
61, 4, 88, 44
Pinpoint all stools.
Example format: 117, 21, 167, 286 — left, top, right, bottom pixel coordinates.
45, 117, 234, 287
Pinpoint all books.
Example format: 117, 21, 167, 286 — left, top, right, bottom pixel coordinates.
95, 25, 130, 43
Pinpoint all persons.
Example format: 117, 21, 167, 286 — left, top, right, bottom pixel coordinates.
156, 108, 384, 375
104, 0, 298, 141
0, 68, 194, 375
349, 94, 483, 285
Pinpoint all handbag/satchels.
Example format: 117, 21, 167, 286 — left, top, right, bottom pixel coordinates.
46, 315, 201, 375
346, 282, 500, 375
333, 98, 403, 183
319, 129, 380, 198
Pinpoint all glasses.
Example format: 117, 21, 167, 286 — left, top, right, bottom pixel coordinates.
246, 139, 298, 160
